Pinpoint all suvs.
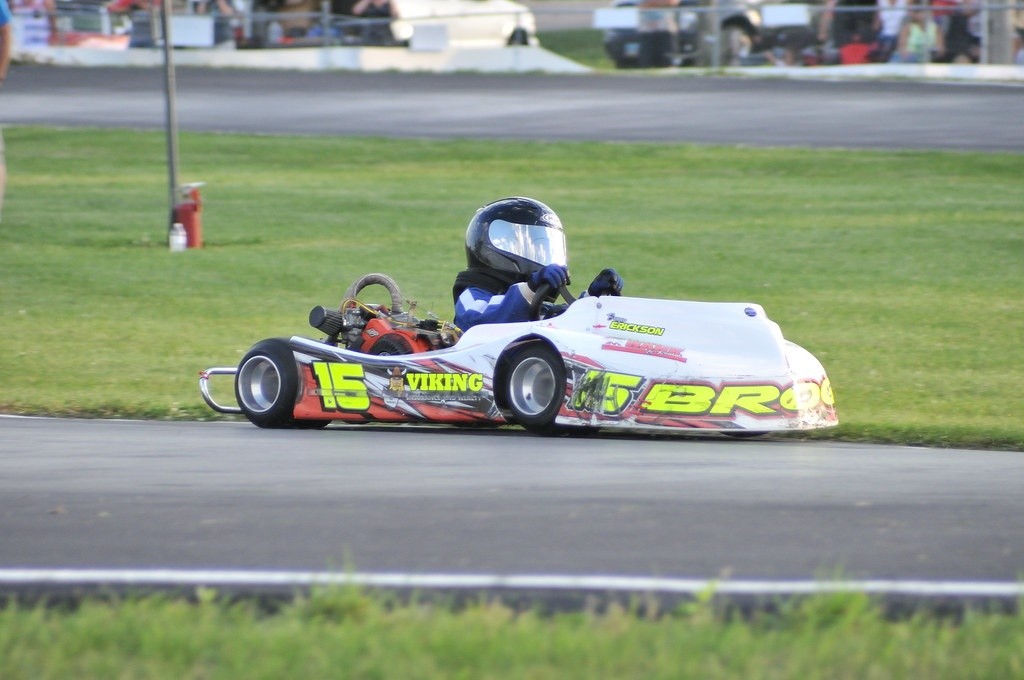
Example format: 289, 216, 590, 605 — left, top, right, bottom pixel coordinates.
242, 0, 539, 53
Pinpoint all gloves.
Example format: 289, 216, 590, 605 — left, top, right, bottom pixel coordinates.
528, 263, 571, 293
588, 269, 623, 298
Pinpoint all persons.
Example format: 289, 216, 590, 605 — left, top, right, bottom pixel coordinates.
351, 0, 400, 46
452, 196, 623, 335
10, 0, 344, 46
706, 0, 1024, 65
0, 0, 13, 198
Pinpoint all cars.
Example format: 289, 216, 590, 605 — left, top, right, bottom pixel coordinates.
599, 1, 815, 68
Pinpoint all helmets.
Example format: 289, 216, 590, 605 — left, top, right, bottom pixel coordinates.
464, 197, 568, 303
